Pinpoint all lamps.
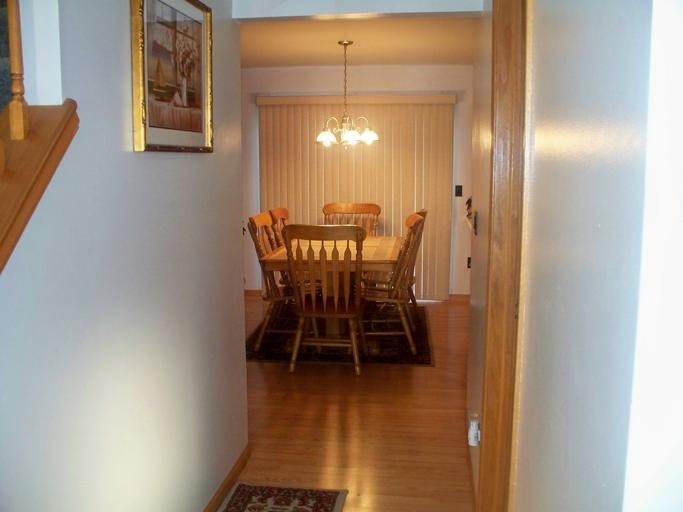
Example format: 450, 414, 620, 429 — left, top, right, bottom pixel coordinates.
314, 38, 380, 147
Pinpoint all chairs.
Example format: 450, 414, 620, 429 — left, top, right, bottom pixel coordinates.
247, 203, 428, 375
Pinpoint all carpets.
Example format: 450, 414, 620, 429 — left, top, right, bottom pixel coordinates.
214, 482, 348, 512
246, 303, 435, 368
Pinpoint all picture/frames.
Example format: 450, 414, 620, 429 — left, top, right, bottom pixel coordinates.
128, 0, 216, 153
